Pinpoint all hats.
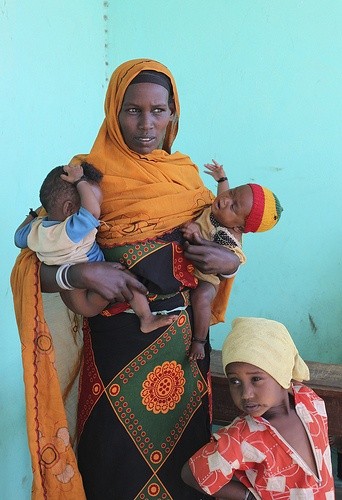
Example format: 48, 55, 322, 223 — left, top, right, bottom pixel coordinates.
242, 182, 284, 234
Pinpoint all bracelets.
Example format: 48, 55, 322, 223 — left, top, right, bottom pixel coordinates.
219, 264, 241, 279
74, 175, 90, 185
55, 262, 75, 292
29, 208, 38, 219
217, 176, 228, 183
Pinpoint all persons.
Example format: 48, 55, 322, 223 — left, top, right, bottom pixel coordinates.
9, 57, 245, 498
180, 317, 336, 499
14, 161, 178, 334
182, 155, 284, 360
